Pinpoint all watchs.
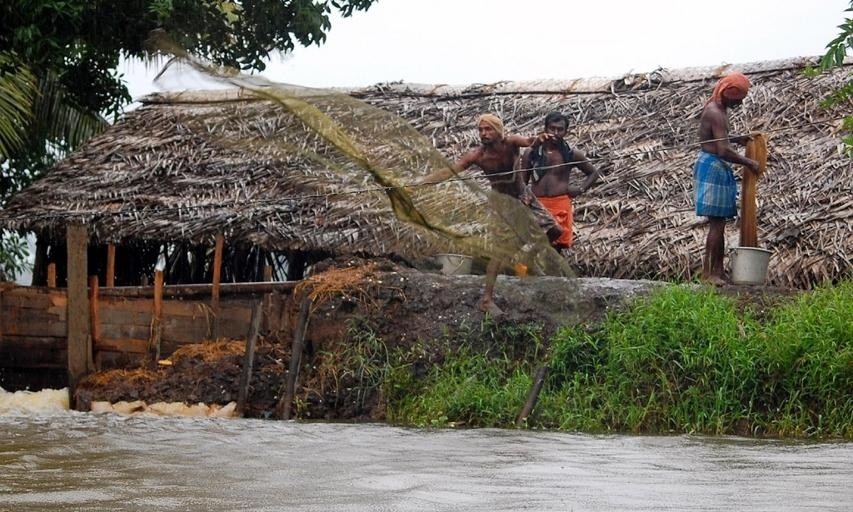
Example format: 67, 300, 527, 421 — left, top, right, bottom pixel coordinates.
578, 184, 584, 193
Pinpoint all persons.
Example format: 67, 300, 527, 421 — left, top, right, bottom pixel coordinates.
403, 113, 564, 319
691, 71, 760, 287
520, 111, 600, 277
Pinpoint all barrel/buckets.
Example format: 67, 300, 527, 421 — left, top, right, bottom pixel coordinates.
435, 254, 474, 274
728, 247, 774, 286
